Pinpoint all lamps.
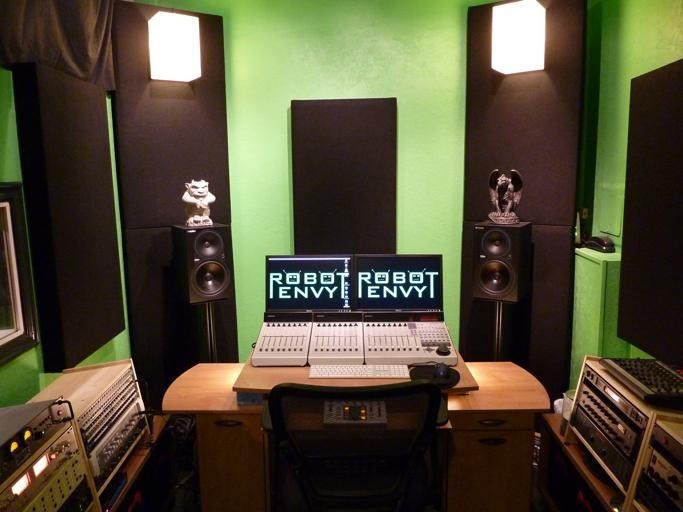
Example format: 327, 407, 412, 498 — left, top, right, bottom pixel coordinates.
489, 1, 547, 75
145, 10, 202, 85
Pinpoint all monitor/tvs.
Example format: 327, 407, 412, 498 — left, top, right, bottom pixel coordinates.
264, 254, 353, 312
353, 254, 442, 312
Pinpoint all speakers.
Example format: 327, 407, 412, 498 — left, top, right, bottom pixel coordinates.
470, 219, 533, 303
170, 222, 234, 304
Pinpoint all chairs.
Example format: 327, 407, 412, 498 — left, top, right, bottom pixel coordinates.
262, 380, 443, 512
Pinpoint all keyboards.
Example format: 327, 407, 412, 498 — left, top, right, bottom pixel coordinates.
307, 364, 410, 378
598, 356, 682, 409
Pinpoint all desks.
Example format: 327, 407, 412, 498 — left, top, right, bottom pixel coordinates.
162, 363, 553, 512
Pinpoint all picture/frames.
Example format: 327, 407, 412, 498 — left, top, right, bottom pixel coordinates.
0, 181, 39, 371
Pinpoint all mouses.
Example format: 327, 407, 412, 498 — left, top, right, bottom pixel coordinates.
434, 362, 449, 378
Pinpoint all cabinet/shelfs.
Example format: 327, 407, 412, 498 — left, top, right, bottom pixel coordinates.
544, 358, 681, 509
91, 411, 178, 509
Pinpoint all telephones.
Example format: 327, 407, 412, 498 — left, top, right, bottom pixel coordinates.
585, 237, 615, 252
